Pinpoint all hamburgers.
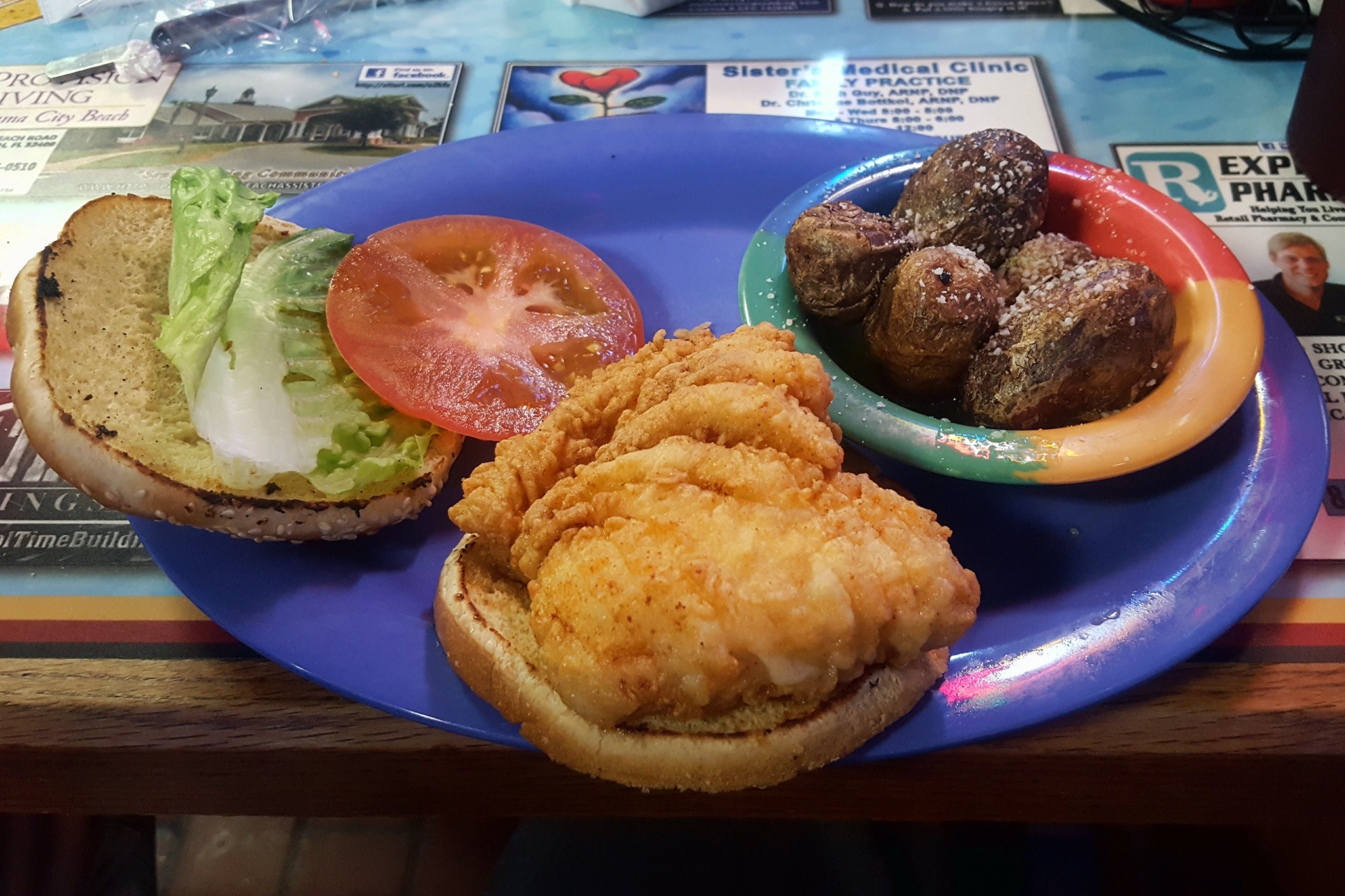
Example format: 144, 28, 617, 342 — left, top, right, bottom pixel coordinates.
7, 165, 980, 796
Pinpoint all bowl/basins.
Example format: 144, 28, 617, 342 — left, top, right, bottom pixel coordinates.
737, 146, 1265, 486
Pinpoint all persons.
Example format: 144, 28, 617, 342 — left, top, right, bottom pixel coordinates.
1250, 231, 1345, 337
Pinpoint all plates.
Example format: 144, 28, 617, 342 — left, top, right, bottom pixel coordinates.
124, 113, 1330, 765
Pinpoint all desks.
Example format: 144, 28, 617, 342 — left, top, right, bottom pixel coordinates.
0, 1, 1344, 895
